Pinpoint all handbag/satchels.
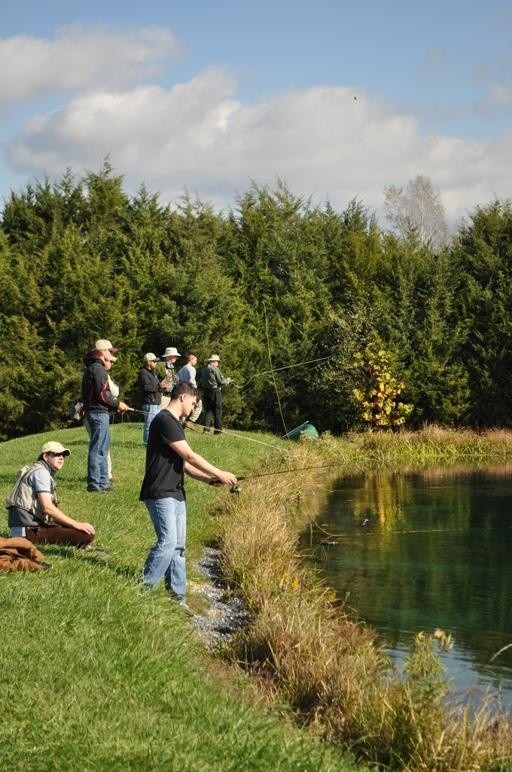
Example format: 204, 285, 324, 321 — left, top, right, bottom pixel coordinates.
63, 400, 87, 422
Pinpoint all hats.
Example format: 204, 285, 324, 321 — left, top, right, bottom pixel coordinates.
208, 354, 221, 361
142, 352, 160, 362
41, 440, 71, 456
109, 355, 118, 362
161, 347, 182, 357
94, 339, 118, 352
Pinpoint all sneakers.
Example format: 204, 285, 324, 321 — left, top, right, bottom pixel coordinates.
169, 598, 195, 617
86, 477, 118, 493
185, 421, 196, 431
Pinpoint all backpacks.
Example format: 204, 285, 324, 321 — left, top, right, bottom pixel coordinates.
108, 375, 120, 399
42, 493, 58, 527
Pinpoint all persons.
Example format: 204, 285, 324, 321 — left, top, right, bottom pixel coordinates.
91, 340, 119, 355
137, 379, 243, 615
81, 349, 132, 495
173, 352, 204, 431
200, 354, 235, 436
139, 352, 170, 449
158, 346, 182, 411
8, 440, 97, 550
100, 349, 120, 482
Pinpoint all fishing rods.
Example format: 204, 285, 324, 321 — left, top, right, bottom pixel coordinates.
228, 357, 329, 384
210, 464, 345, 485
117, 406, 284, 451
